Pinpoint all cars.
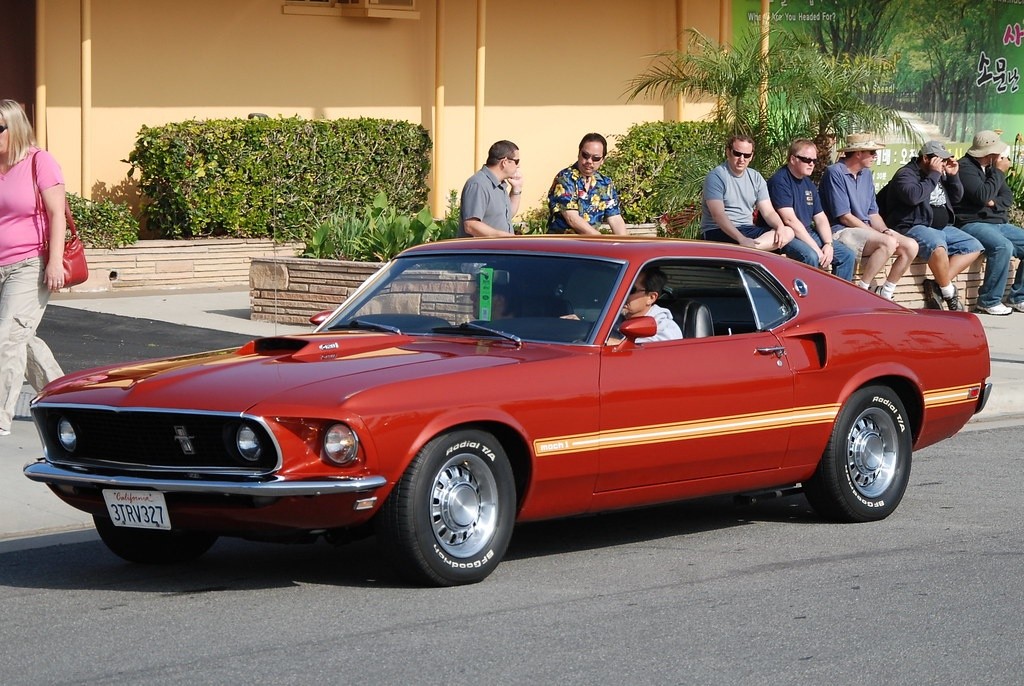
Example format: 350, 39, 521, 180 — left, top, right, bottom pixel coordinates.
22, 235, 992, 590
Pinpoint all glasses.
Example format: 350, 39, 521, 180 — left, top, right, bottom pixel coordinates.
630, 286, 649, 294
0, 126, 8, 134
498, 156, 519, 165
861, 150, 876, 155
730, 148, 752, 158
792, 155, 817, 165
580, 148, 604, 161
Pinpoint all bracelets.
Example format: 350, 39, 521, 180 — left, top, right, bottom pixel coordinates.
511, 190, 522, 195
882, 229, 890, 234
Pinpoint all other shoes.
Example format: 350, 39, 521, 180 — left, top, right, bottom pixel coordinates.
873, 285, 893, 302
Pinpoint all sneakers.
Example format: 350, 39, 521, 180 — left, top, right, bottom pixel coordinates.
923, 279, 944, 312
1006, 299, 1024, 312
977, 299, 1012, 315
946, 285, 964, 313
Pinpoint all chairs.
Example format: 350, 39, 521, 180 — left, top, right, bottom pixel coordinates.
657, 288, 777, 336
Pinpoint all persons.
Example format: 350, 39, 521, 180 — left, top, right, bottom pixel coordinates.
756, 138, 856, 287
948, 130, 1024, 316
559, 264, 683, 345
470, 264, 529, 321
699, 134, 794, 253
458, 139, 523, 237
546, 133, 630, 236
876, 140, 985, 312
819, 132, 919, 304
0, 100, 66, 435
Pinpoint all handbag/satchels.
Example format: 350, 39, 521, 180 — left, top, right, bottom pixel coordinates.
41, 236, 88, 289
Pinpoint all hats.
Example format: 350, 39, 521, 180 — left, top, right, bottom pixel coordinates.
837, 132, 884, 152
918, 141, 954, 159
967, 130, 1007, 157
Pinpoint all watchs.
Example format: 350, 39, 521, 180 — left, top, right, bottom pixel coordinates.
824, 242, 834, 247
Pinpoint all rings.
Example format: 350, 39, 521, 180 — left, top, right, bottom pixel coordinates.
57, 280, 61, 283
